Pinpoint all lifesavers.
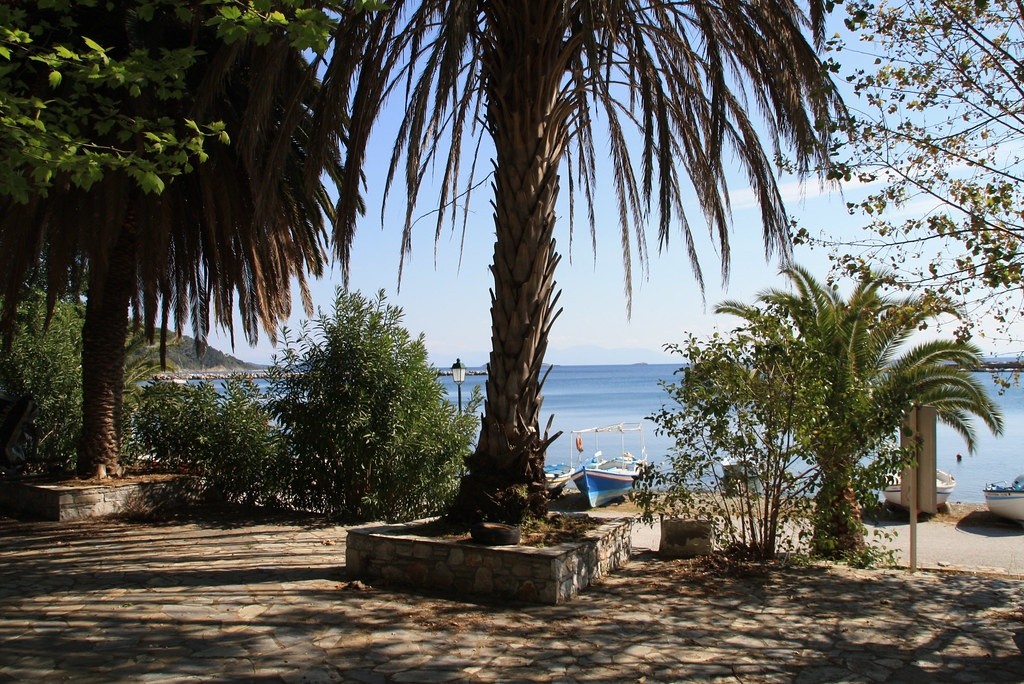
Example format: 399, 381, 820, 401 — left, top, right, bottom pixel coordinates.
576, 435, 583, 452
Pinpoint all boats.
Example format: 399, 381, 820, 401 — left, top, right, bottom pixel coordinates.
722, 452, 765, 480
986, 477, 1024, 522
572, 452, 649, 506
883, 465, 955, 511
544, 462, 575, 495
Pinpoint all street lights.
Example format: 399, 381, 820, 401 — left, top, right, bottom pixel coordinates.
452, 359, 467, 415
